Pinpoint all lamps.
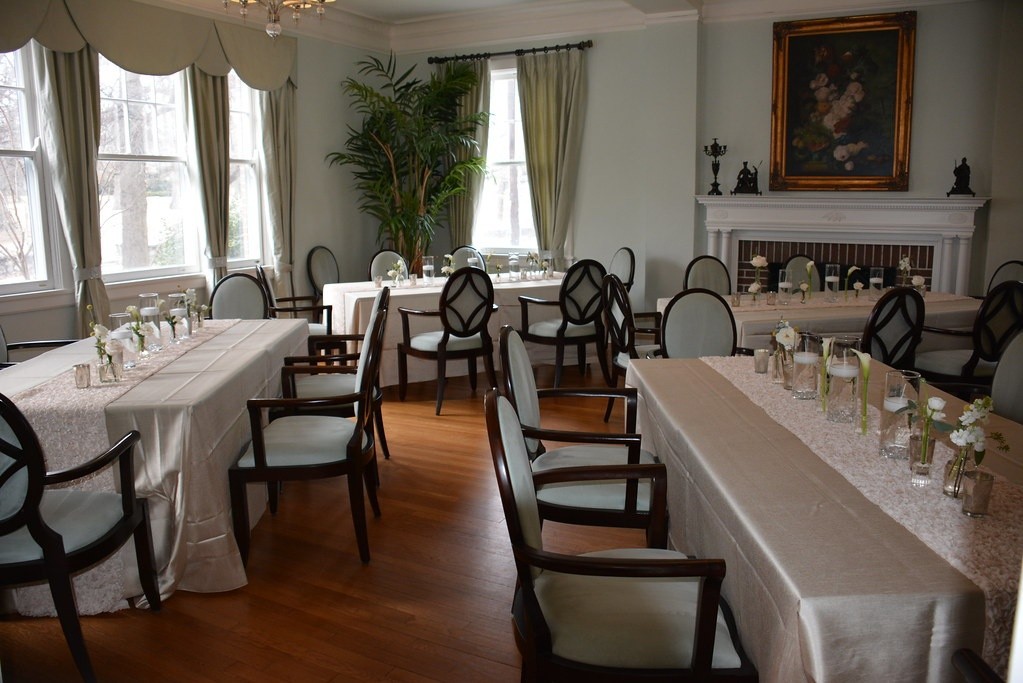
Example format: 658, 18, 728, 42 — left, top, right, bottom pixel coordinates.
217, 0, 334, 36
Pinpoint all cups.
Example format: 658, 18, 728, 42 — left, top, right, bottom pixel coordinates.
879, 370, 921, 460
169, 293, 189, 338
467, 250, 478, 267
791, 332, 820, 400
509, 252, 520, 281
73, 364, 90, 388
962, 471, 993, 517
422, 256, 435, 284
754, 349, 769, 373
869, 268, 884, 302
826, 336, 862, 423
825, 265, 840, 304
109, 312, 135, 368
136, 292, 161, 351
778, 269, 792, 304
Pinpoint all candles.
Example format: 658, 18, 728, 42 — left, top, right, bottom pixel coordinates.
509, 260, 517, 265
885, 385, 914, 413
138, 299, 158, 315
825, 270, 841, 282
467, 253, 477, 262
171, 299, 187, 314
423, 260, 433, 271
112, 321, 131, 340
780, 276, 792, 288
871, 271, 884, 282
794, 343, 818, 362
825, 348, 859, 375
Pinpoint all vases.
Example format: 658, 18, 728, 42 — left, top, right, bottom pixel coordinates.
396, 272, 927, 309
775, 352, 965, 494
96, 308, 207, 385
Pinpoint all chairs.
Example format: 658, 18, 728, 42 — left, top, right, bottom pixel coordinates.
971, 260, 1023, 301
195, 247, 822, 682
602, 274, 660, 424
797, 290, 925, 385
916, 279, 1023, 385
0, 331, 164, 682
926, 328, 1023, 426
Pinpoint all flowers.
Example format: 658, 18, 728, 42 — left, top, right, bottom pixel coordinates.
769, 321, 1006, 452
83, 288, 206, 360
385, 254, 924, 292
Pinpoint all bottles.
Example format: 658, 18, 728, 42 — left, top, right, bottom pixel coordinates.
730, 292, 740, 306
766, 291, 776, 305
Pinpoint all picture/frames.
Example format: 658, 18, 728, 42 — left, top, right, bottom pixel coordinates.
764, 10, 917, 191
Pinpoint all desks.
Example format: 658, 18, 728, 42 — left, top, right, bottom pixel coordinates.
322, 272, 595, 389
655, 289, 981, 357
627, 356, 1023, 683
1, 314, 310, 618
